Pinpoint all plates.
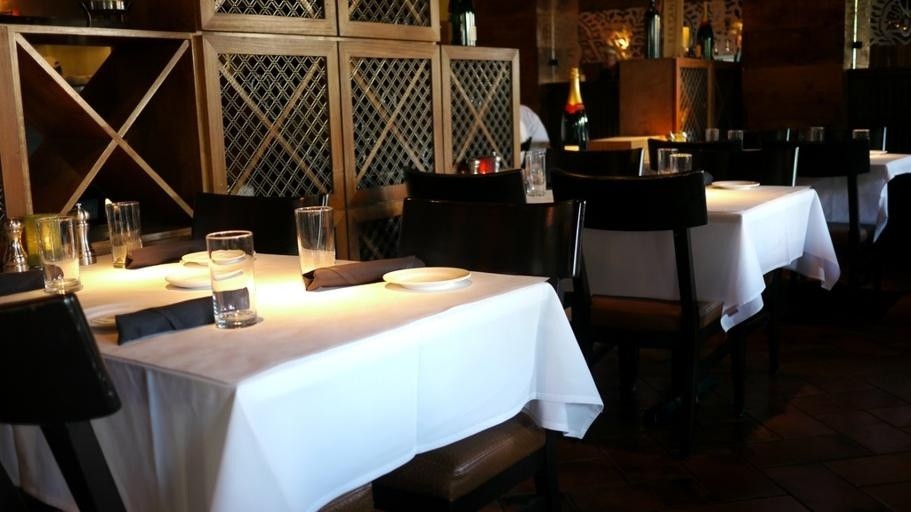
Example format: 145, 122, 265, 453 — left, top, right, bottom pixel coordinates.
710, 180, 760, 189
81, 302, 153, 331
181, 250, 246, 265
383, 268, 471, 289
165, 267, 210, 289
869, 150, 887, 155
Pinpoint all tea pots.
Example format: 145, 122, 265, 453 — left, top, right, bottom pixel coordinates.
798, 126, 824, 142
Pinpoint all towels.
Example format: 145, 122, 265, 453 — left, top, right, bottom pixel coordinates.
302, 254, 425, 291
115, 296, 216, 345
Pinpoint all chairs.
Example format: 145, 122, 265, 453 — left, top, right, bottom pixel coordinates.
192, 193, 303, 256
402, 165, 527, 206
545, 147, 643, 190
547, 165, 729, 442
882, 126, 910, 155
373, 196, 587, 511
648, 138, 736, 181
1, 268, 372, 512
755, 140, 876, 285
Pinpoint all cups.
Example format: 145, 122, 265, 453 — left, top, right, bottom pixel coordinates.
853, 128, 870, 142
525, 152, 545, 196
727, 130, 743, 150
667, 154, 692, 174
656, 148, 677, 175
36, 215, 80, 293
295, 206, 335, 272
205, 229, 257, 329
104, 200, 142, 268
705, 127, 719, 141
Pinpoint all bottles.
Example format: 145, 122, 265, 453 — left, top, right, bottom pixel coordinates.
696, 0, 713, 60
560, 67, 588, 145
69, 201, 96, 265
447, 0, 477, 45
1, 217, 30, 266
642, 0, 661, 59
466, 154, 500, 175
